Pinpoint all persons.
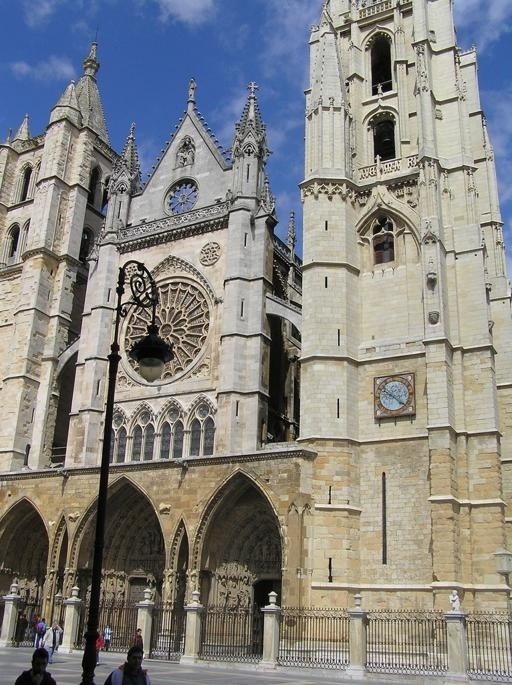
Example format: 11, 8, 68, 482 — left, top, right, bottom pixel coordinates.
129, 629, 143, 654
104, 647, 152, 685
101, 625, 113, 651
14, 647, 57, 685
16, 613, 64, 665
94, 625, 105, 667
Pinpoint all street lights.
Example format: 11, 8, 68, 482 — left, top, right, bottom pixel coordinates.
77, 260, 175, 685
